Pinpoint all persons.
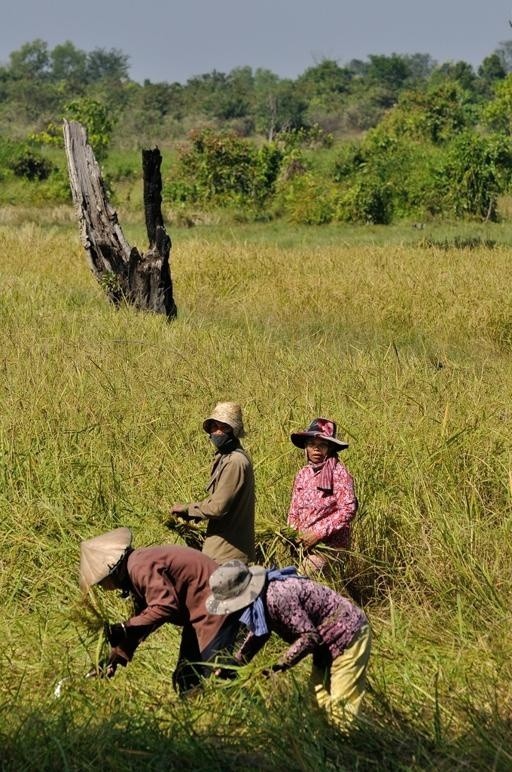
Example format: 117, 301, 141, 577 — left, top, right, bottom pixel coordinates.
286, 419, 357, 578
169, 401, 254, 565
205, 558, 371, 734
80, 527, 238, 697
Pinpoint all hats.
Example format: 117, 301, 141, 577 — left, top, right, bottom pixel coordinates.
202, 401, 243, 438
205, 559, 267, 616
79, 526, 132, 588
291, 418, 348, 452
